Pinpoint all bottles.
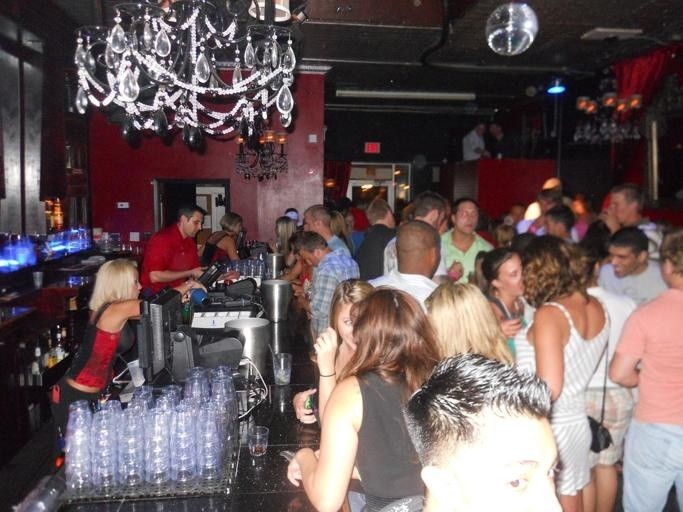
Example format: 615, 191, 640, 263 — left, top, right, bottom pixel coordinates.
32, 339, 42, 374
50, 324, 65, 363
61, 328, 69, 357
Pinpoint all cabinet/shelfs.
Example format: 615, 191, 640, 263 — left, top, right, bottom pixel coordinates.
0, 272, 94, 473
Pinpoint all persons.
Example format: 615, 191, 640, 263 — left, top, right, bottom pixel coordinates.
141, 205, 206, 297
198, 213, 243, 265
51, 258, 207, 446
270, 123, 683, 511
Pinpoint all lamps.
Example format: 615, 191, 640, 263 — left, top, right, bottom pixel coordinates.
574, 29, 642, 145
234, 127, 288, 181
547, 78, 566, 94
485, 3, 538, 56
74, 0, 297, 155
249, 0, 291, 22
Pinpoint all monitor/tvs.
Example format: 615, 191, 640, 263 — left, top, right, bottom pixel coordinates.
136, 286, 194, 384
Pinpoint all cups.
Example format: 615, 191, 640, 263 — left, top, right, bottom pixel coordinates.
32, 272, 44, 290
231, 257, 264, 277
273, 352, 292, 386
246, 426, 271, 457
126, 360, 145, 386
64, 366, 237, 495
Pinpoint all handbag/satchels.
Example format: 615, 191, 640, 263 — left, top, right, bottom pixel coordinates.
588, 415, 614, 453
